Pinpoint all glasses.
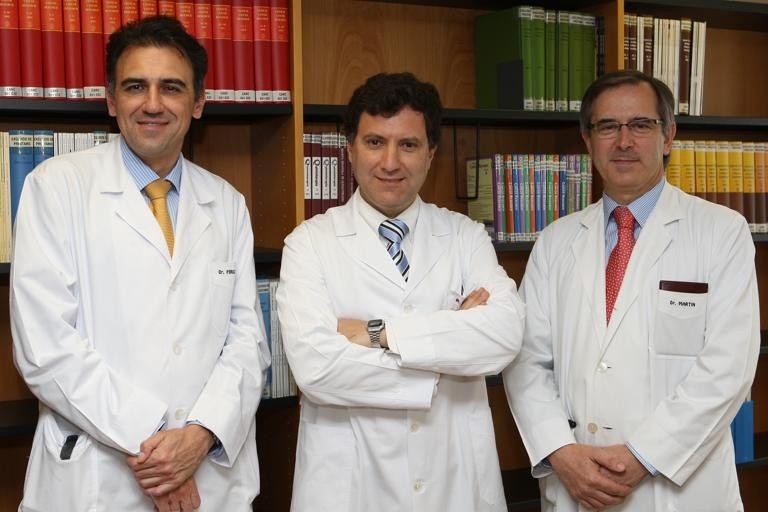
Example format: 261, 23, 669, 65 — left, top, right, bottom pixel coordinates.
592, 119, 664, 139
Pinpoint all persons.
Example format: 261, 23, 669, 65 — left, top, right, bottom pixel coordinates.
9, 15, 272, 512
277, 72, 527, 511
502, 71, 764, 512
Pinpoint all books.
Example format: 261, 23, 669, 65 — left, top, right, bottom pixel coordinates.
657, 138, 768, 227
1, 1, 294, 91
460, 151, 598, 244
462, 6, 709, 117
253, 278, 302, 398
2, 129, 126, 265
303, 124, 364, 223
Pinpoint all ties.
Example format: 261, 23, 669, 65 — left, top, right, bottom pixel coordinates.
379, 219, 409, 281
143, 179, 174, 257
606, 206, 636, 321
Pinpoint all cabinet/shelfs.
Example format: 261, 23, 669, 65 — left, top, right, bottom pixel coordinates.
0, 0, 307, 432
298, 0, 768, 510
